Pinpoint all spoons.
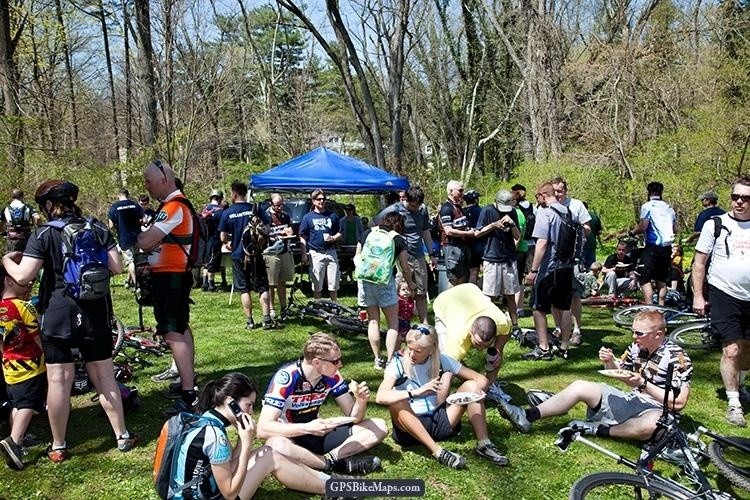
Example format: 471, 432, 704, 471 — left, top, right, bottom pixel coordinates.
608, 352, 622, 362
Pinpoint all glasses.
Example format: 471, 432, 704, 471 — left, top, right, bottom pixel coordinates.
152, 160, 167, 183
631, 328, 654, 338
315, 198, 326, 202
412, 325, 431, 336
314, 356, 342, 365
730, 194, 750, 202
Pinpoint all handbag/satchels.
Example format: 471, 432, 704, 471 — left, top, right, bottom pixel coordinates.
513, 327, 559, 348
134, 252, 159, 306
687, 217, 722, 316
91, 386, 138, 417
653, 290, 681, 305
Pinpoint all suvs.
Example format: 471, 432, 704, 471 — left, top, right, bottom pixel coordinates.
258, 196, 369, 265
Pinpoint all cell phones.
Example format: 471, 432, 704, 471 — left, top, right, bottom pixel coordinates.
227, 399, 249, 429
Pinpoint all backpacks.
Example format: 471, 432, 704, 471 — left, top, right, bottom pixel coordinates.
517, 203, 536, 241
8, 204, 27, 229
156, 197, 212, 268
201, 205, 224, 225
43, 214, 111, 300
153, 413, 228, 499
550, 205, 588, 266
241, 203, 269, 257
354, 225, 400, 286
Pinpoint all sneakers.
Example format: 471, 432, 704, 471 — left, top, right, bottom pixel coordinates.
160, 394, 200, 420
498, 400, 533, 434
151, 369, 179, 383
162, 374, 200, 398
46, 441, 67, 462
0, 432, 41, 471
523, 347, 553, 361
244, 314, 284, 330
726, 385, 750, 427
437, 449, 466, 470
484, 381, 513, 403
476, 443, 509, 466
569, 420, 609, 438
552, 328, 583, 360
116, 432, 141, 451
375, 355, 386, 369
337, 455, 381, 476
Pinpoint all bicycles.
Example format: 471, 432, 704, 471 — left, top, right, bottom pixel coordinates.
281, 275, 386, 338
613, 300, 716, 353
110, 313, 169, 365
577, 287, 646, 306
551, 360, 750, 500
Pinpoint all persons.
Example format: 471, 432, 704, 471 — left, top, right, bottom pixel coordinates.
0, 157, 750, 500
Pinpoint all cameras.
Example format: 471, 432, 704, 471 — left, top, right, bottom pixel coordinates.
503, 222, 515, 227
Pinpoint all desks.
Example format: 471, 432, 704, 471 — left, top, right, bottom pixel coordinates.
221, 250, 359, 306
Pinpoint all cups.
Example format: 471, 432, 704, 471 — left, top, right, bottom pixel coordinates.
323, 233, 330, 241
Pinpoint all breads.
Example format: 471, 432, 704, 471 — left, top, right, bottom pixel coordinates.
349, 380, 364, 400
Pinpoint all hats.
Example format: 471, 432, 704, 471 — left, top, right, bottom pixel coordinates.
496, 190, 512, 212
699, 192, 718, 199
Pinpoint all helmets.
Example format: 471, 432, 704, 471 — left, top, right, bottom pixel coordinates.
35, 180, 79, 204
527, 390, 555, 407
209, 189, 223, 200
463, 190, 481, 199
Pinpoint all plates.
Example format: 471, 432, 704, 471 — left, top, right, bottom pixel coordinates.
597, 369, 633, 378
444, 392, 486, 405
280, 235, 297, 240
321, 416, 357, 426
